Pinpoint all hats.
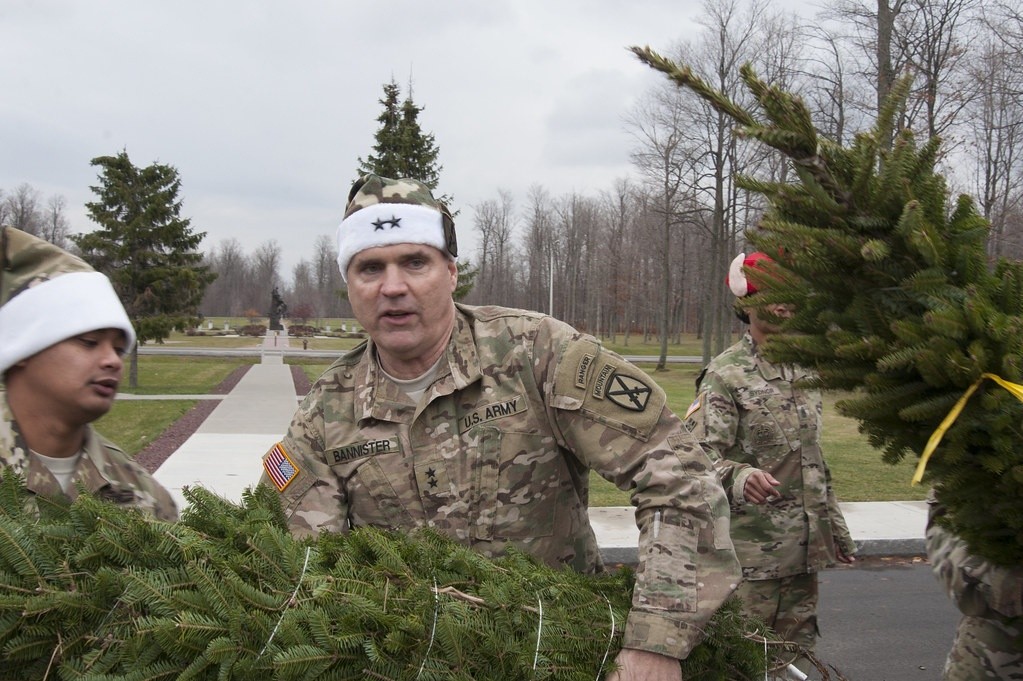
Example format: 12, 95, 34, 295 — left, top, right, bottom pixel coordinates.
335, 172, 459, 283
726, 241, 793, 299
0, 221, 137, 378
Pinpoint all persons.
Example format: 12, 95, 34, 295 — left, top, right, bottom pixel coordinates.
685, 251, 858, 681
0, 225, 178, 523
259, 176, 742, 681
926, 480, 1023, 681
